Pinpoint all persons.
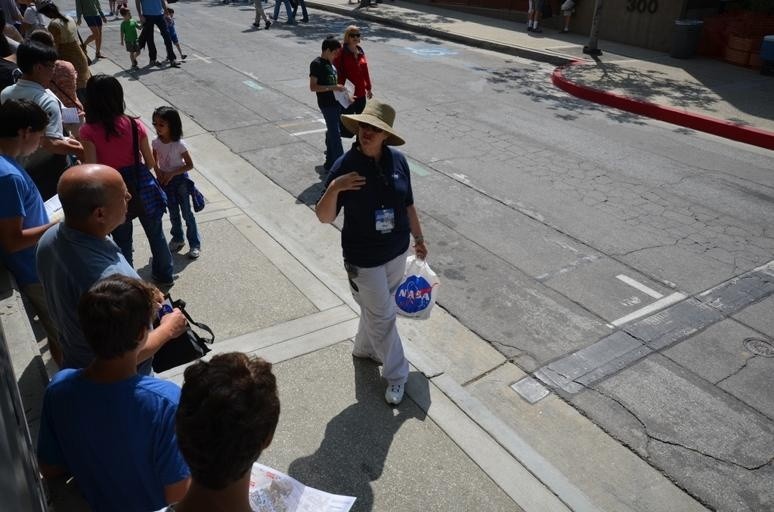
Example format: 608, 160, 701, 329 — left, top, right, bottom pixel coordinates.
527, 0, 543, 33
309, 35, 358, 171
0, 0, 281, 512
313, 97, 441, 407
325, 25, 374, 146
253, 0, 309, 30
558, 0, 579, 33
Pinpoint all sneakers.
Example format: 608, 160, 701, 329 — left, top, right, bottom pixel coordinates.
352, 345, 385, 364
169, 240, 200, 258
152, 272, 174, 287
385, 381, 405, 407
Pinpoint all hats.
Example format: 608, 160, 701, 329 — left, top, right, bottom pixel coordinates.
340, 99, 406, 145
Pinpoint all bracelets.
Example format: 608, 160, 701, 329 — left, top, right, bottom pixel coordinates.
415, 235, 424, 246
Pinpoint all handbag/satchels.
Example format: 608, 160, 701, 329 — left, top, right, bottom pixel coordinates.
125, 189, 147, 221
81, 43, 97, 65
150, 293, 215, 374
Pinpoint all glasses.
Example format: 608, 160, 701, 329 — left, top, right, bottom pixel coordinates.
153, 121, 166, 127
357, 121, 382, 133
349, 32, 360, 37
